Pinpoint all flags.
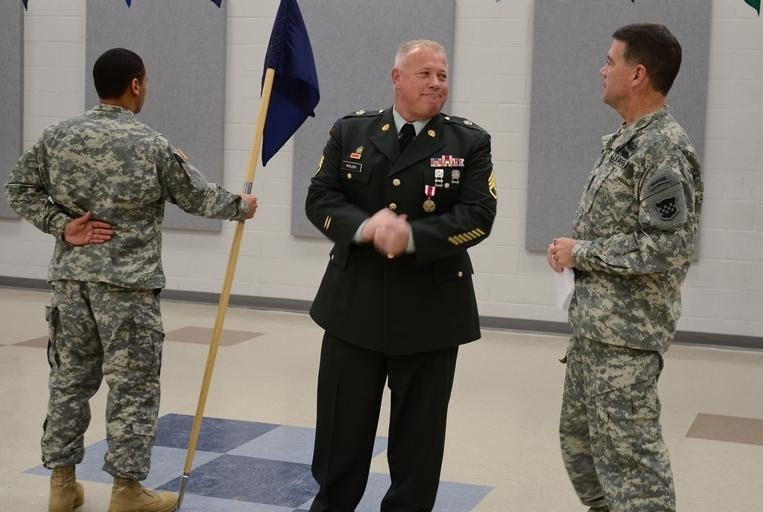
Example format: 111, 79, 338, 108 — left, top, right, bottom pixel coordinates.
252, 0, 321, 169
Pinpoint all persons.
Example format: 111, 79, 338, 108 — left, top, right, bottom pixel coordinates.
2, 46, 257, 511
300, 40, 495, 510
543, 23, 704, 512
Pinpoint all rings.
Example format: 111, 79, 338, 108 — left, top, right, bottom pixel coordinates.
551, 255, 555, 263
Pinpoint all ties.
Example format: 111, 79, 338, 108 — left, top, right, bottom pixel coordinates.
395, 124, 418, 155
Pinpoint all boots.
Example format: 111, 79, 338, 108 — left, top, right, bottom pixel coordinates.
108, 478, 179, 512
49, 466, 84, 512
